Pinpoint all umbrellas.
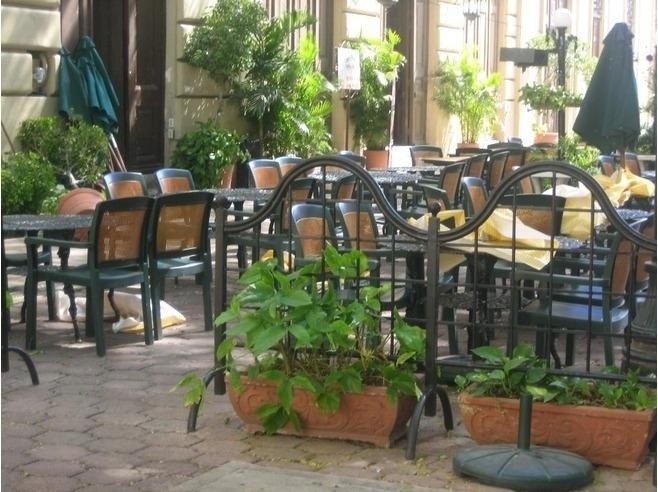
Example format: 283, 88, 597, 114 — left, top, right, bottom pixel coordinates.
571, 21, 645, 175
54, 34, 126, 174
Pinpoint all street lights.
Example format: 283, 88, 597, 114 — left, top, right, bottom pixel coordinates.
548, 5, 573, 161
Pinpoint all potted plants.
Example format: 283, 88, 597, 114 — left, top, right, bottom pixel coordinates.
455, 341, 656, 471
171, 240, 426, 452
167, 119, 247, 190
432, 41, 572, 146
169, 240, 657, 472
336, 25, 409, 171
